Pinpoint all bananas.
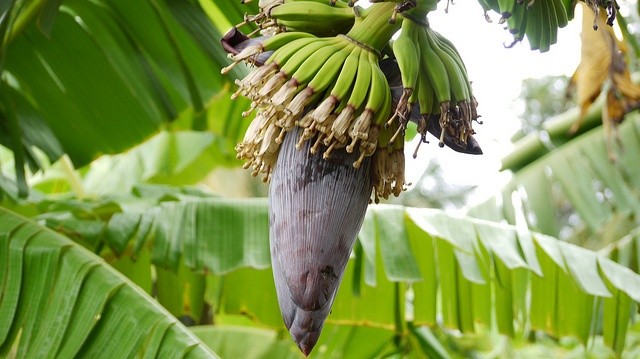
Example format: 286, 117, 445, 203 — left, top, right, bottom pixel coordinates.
220, 0, 483, 204
477, 1, 621, 53
